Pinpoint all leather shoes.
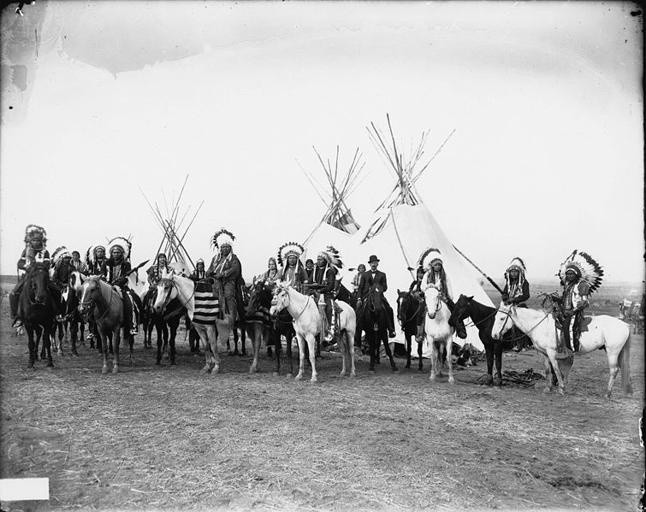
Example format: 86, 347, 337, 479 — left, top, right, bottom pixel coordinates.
390, 330, 396, 337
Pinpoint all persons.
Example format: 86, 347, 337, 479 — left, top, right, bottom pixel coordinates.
415, 245, 467, 342
8, 224, 136, 337
356, 255, 396, 338
503, 257, 530, 351
410, 267, 424, 293
552, 249, 604, 352
193, 259, 207, 279
206, 243, 241, 323
260, 241, 340, 343
353, 264, 366, 286
142, 253, 174, 311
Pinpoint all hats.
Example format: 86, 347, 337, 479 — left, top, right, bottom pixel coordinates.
51, 247, 71, 269
419, 248, 442, 271
506, 257, 526, 277
86, 237, 131, 266
24, 224, 47, 247
277, 242, 304, 265
559, 250, 603, 296
368, 255, 380, 263
318, 245, 343, 269
213, 229, 235, 254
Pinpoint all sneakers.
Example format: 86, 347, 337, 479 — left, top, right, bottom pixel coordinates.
132, 325, 138, 335
458, 331, 466, 338
13, 320, 24, 327
217, 315, 234, 328
56, 315, 66, 322
87, 332, 98, 340
555, 347, 575, 360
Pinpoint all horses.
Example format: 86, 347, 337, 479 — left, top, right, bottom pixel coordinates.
17, 249, 635, 399
